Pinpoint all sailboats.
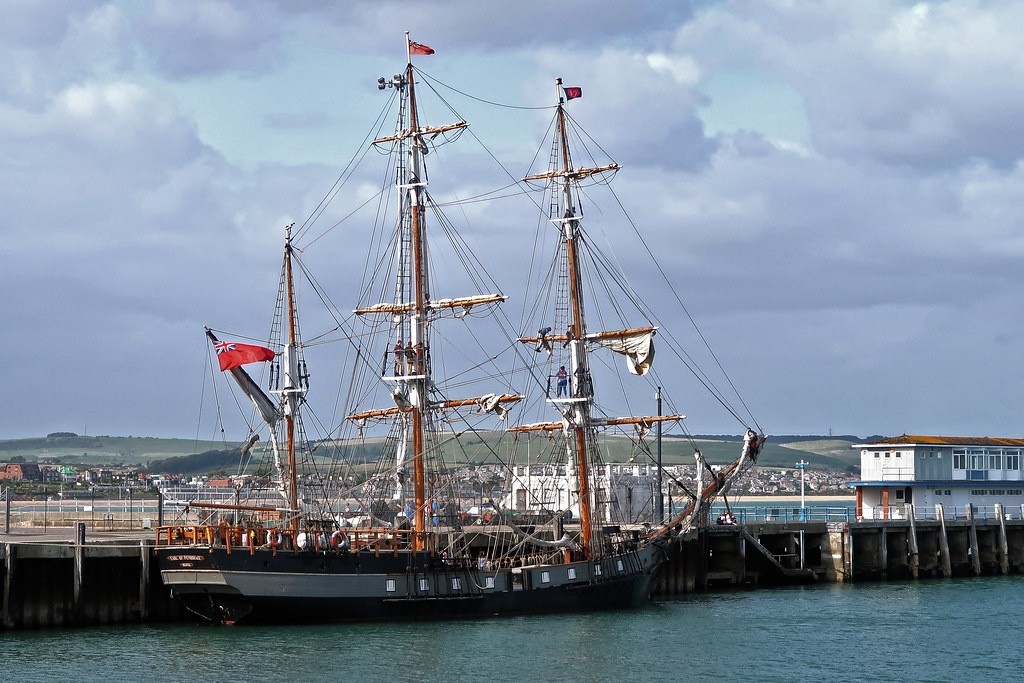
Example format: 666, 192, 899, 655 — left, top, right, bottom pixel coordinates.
135, 29, 770, 628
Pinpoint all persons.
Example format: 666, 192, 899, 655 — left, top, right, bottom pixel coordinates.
393, 340, 405, 376
556, 366, 567, 399
717, 512, 737, 525
413, 341, 425, 374
574, 363, 586, 398
535, 327, 551, 352
405, 341, 417, 376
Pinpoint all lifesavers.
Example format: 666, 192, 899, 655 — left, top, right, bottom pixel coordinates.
332, 530, 346, 547
266, 529, 282, 546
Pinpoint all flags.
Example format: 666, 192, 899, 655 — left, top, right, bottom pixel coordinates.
409, 39, 435, 55
563, 87, 582, 101
213, 341, 275, 372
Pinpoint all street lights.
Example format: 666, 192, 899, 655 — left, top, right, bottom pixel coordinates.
654, 387, 666, 523
794, 459, 811, 522
